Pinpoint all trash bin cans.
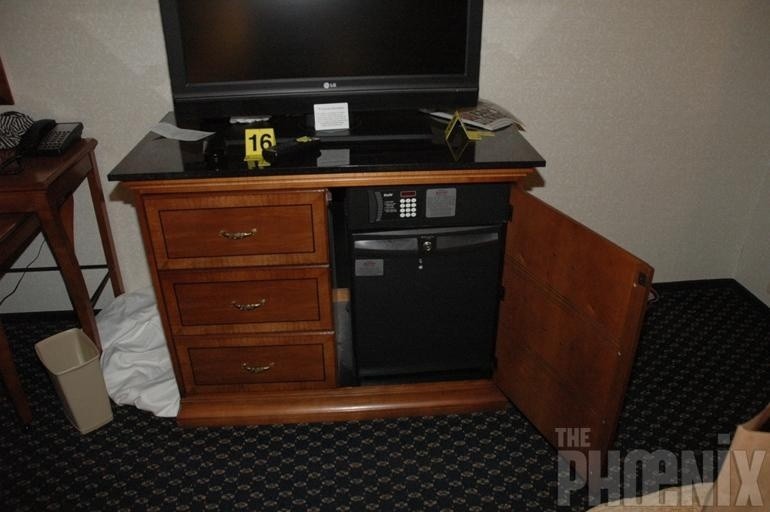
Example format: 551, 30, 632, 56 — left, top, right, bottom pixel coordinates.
34, 327, 115, 435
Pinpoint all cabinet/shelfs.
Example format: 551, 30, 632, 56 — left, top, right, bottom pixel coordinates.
107, 109, 649, 490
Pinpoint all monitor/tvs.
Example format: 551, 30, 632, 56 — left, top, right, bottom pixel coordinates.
156, 1, 486, 136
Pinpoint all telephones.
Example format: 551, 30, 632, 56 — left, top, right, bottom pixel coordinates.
16, 119, 84, 156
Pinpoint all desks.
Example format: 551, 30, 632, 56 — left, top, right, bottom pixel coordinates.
0, 138, 126, 434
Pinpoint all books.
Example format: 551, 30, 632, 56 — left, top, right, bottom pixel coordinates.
431, 97, 528, 131
